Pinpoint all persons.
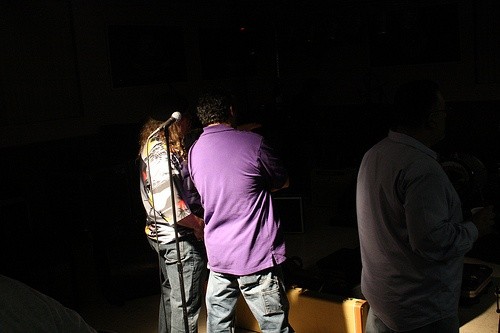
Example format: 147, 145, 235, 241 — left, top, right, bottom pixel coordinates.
186, 74, 293, 333
355, 80, 500, 333
137, 90, 209, 333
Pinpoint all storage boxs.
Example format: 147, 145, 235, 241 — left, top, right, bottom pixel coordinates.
272, 195, 305, 235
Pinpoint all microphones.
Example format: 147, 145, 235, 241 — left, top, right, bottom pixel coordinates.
148, 112, 182, 139
